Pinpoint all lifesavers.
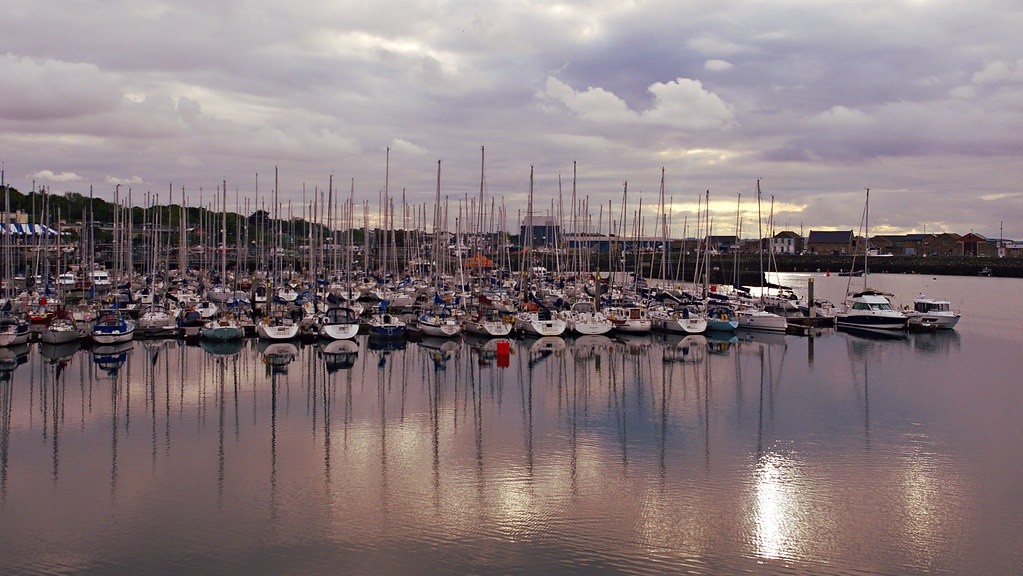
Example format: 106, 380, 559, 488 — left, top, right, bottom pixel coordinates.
323, 316, 330, 324
190, 306, 195, 310
722, 343, 727, 350
720, 313, 728, 320
740, 306, 747, 311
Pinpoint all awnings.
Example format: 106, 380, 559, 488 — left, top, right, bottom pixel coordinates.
0, 223, 71, 236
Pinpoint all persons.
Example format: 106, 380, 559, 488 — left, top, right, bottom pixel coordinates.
38, 294, 47, 314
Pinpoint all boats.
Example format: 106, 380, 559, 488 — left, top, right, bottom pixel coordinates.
41, 304, 79, 344
255, 304, 300, 339
839, 270, 864, 277
699, 300, 739, 330
829, 186, 961, 329
418, 311, 463, 336
602, 300, 652, 331
464, 309, 512, 335
317, 306, 360, 339
91, 305, 135, 344
558, 302, 612, 334
978, 267, 992, 277
0, 266, 799, 335
198, 310, 245, 340
514, 310, 566, 335
0, 316, 30, 346
646, 298, 707, 332
734, 301, 788, 330
764, 305, 838, 327
369, 314, 406, 337
